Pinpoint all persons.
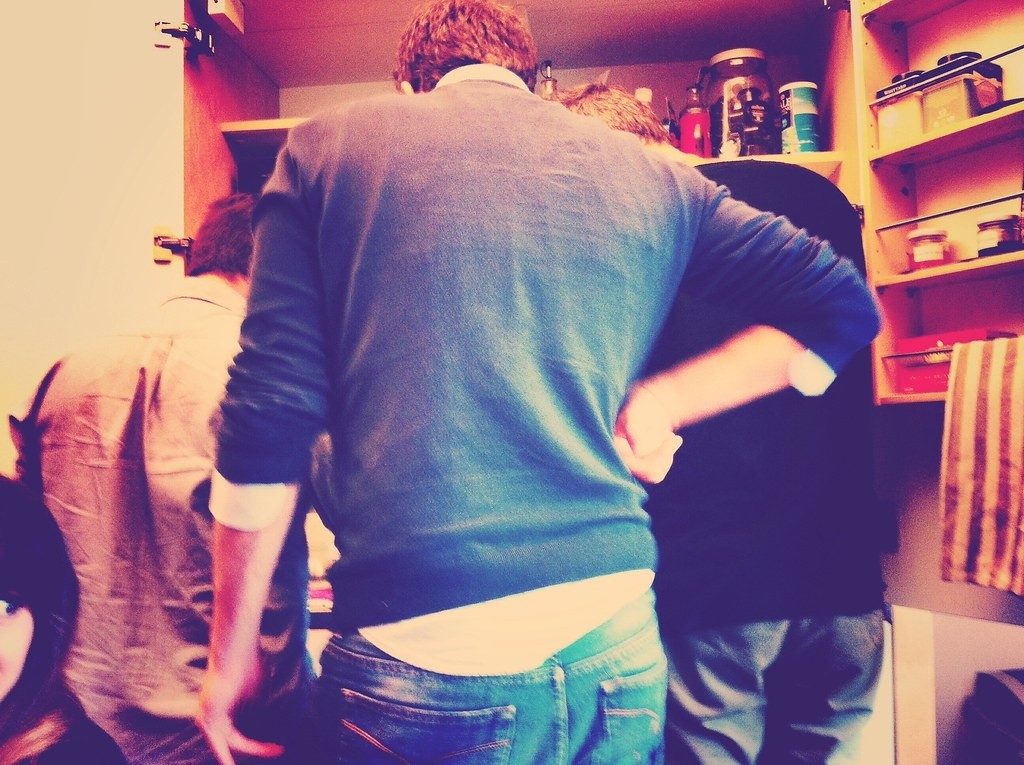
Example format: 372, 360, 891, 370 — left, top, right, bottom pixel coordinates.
544, 85, 884, 765
0, 188, 337, 765
196, 0, 882, 765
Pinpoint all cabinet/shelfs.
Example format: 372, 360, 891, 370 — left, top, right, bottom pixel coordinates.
849, 0, 1024, 408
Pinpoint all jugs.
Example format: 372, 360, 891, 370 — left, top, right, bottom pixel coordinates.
697, 48, 779, 158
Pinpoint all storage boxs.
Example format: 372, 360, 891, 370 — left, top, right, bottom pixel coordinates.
869, 55, 1002, 148
896, 327, 1019, 395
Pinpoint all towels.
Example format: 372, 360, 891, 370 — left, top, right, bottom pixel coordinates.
939, 335, 1024, 598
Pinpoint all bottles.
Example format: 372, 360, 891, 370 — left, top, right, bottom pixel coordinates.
634, 88, 651, 106
679, 85, 712, 158
